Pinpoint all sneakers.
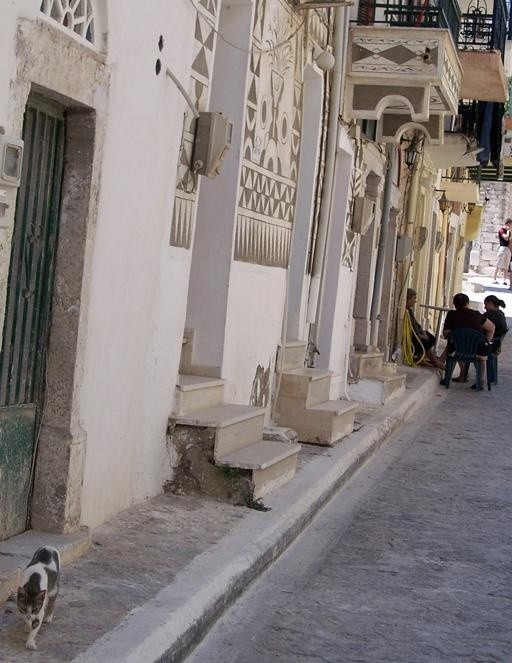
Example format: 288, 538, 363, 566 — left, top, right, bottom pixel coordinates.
440, 373, 484, 391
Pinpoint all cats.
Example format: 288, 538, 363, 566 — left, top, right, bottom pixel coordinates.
15, 546, 61, 650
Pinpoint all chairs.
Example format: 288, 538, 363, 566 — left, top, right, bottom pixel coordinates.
441, 326, 511, 392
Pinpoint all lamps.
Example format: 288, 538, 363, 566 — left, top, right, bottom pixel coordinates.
399, 130, 423, 169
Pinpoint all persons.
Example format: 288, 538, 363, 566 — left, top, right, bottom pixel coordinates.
451, 293, 508, 382
437, 291, 495, 390
402, 287, 445, 370
492, 217, 512, 285
